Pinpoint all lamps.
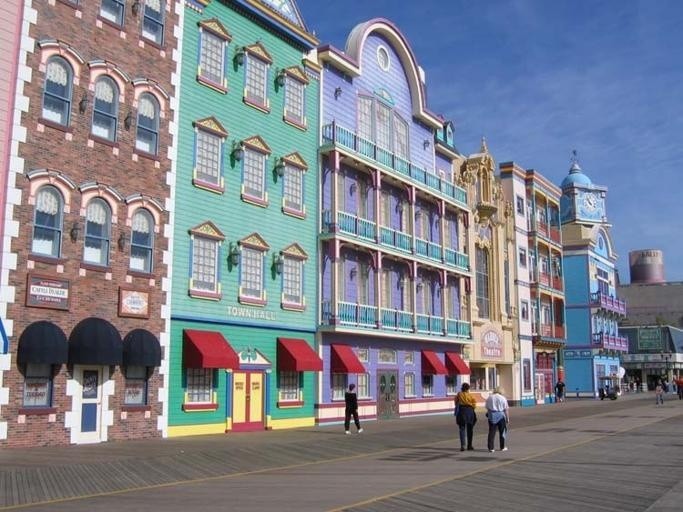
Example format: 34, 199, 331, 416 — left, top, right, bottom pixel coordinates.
233, 45, 286, 86
231, 242, 283, 276
230, 139, 285, 178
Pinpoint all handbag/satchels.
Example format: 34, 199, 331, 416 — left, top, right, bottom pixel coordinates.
453, 392, 462, 416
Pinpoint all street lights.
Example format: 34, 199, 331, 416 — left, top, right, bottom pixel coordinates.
661, 349, 672, 382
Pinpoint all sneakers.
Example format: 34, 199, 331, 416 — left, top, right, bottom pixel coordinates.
357, 427, 363, 433
344, 430, 351, 435
489, 449, 496, 452
502, 447, 508, 451
460, 446, 473, 451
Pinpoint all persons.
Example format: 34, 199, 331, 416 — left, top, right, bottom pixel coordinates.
455, 383, 477, 451
557, 378, 565, 402
486, 386, 509, 452
344, 384, 363, 434
655, 374, 683, 404
636, 377, 641, 392
